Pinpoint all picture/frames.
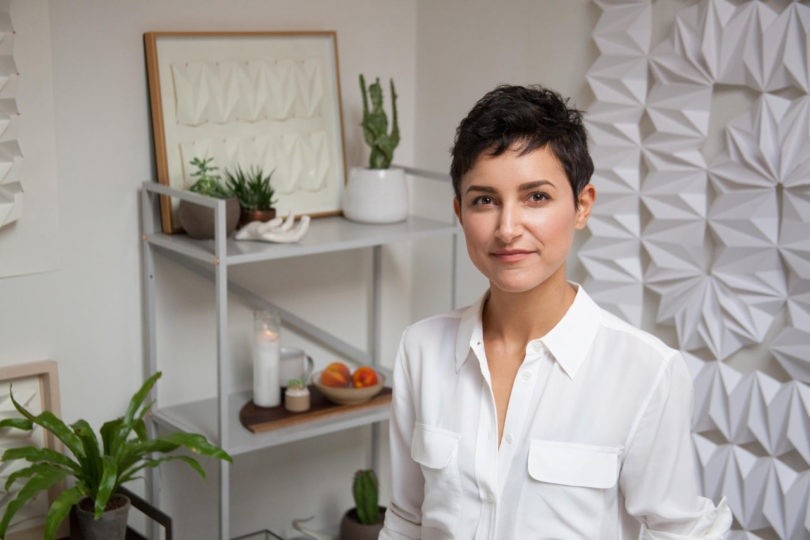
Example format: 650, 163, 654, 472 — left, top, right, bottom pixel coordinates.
145, 30, 347, 234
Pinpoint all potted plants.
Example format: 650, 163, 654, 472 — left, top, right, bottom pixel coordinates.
0, 370, 233, 540
180, 158, 241, 239
341, 470, 386, 540
226, 164, 276, 229
342, 74, 410, 225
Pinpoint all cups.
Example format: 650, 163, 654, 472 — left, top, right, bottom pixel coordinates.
280, 345, 314, 388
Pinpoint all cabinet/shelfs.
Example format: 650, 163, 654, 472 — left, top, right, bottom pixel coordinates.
142, 165, 462, 540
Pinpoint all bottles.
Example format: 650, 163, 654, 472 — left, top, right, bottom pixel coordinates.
251, 308, 280, 407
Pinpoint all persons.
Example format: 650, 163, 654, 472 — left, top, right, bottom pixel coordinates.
375, 83, 734, 539
234, 211, 311, 243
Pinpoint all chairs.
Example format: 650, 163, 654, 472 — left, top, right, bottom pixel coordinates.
0, 359, 173, 540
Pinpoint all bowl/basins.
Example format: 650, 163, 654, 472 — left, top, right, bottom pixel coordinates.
312, 369, 386, 404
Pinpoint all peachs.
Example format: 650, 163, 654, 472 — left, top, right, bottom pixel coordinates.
352, 367, 377, 389
322, 363, 350, 386
